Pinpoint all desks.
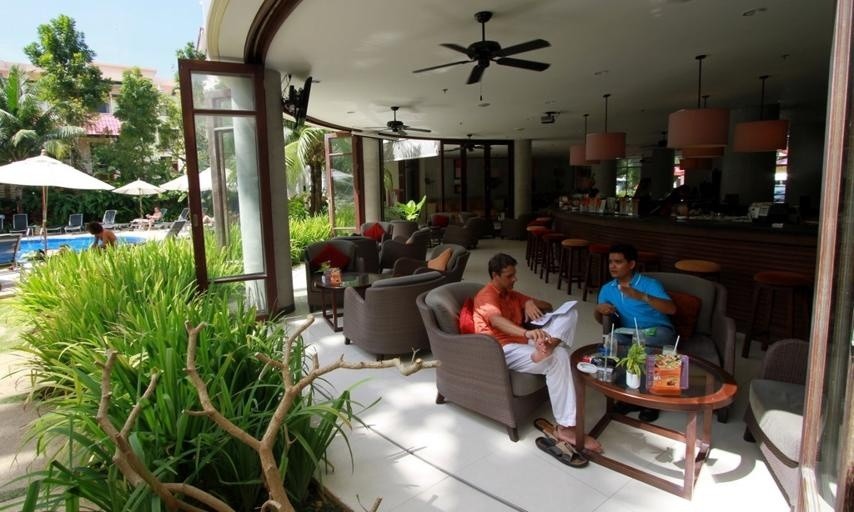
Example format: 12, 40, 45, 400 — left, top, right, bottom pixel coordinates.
566, 339, 740, 499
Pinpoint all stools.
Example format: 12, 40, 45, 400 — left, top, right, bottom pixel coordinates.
675, 260, 720, 278
556, 240, 588, 293
582, 244, 612, 302
634, 252, 655, 268
525, 216, 568, 284
741, 271, 811, 359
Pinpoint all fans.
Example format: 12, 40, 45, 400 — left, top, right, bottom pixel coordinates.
352, 106, 431, 140
441, 133, 491, 154
410, 9, 552, 88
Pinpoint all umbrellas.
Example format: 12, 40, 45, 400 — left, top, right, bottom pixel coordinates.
159, 174, 210, 219
202, 166, 233, 179
0, 150, 114, 239
110, 178, 165, 218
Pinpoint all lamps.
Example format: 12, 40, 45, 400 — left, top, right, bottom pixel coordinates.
679, 94, 725, 159
582, 93, 627, 162
664, 55, 730, 149
733, 76, 789, 153
568, 112, 601, 168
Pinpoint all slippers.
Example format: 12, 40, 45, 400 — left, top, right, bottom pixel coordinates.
534, 417, 588, 467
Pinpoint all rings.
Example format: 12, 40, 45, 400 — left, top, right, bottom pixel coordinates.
605, 309, 608, 314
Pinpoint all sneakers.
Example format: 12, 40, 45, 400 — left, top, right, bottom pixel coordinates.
616, 400, 659, 422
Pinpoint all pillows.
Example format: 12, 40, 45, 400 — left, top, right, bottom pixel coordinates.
457, 293, 478, 335
663, 287, 703, 340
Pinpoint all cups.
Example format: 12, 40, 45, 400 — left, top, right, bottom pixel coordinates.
605, 334, 618, 355
663, 345, 677, 356
631, 330, 646, 348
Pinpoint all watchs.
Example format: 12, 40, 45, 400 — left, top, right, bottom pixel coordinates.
643, 291, 651, 302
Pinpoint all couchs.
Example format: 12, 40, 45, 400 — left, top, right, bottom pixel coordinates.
601, 269, 739, 423
741, 337, 810, 512
413, 277, 555, 442
300, 208, 535, 362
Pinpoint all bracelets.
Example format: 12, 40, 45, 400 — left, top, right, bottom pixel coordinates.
522, 329, 528, 338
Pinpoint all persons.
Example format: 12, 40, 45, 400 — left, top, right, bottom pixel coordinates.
634, 177, 653, 207
202, 213, 214, 224
87, 224, 117, 251
578, 177, 599, 198
145, 207, 162, 231
593, 241, 678, 423
474, 254, 603, 453
